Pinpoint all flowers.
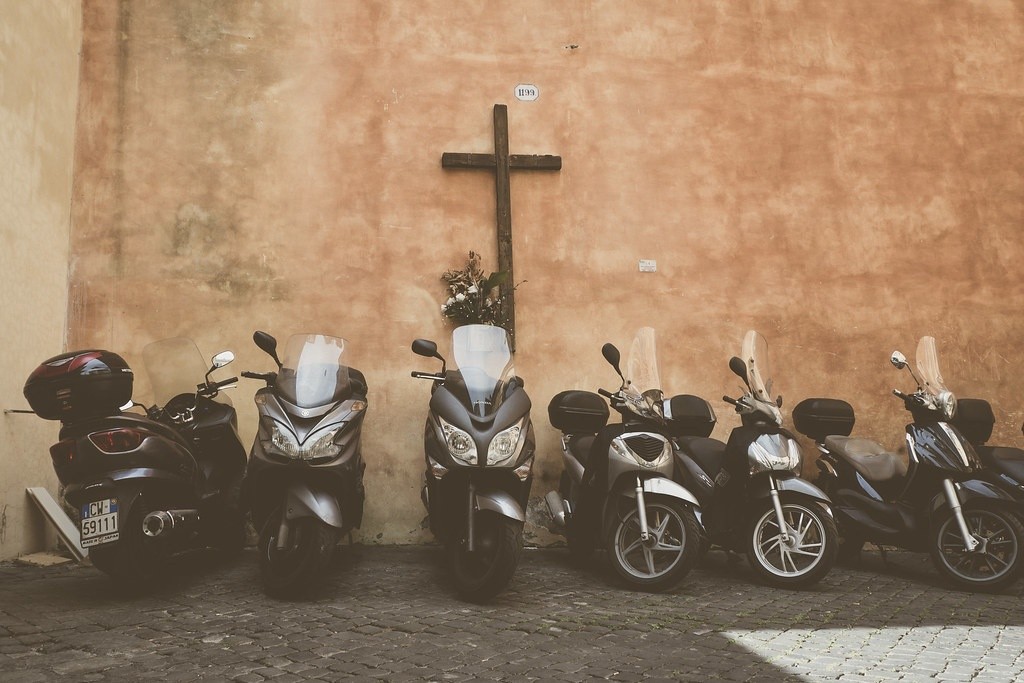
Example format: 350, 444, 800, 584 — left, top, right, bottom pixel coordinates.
440, 250, 529, 340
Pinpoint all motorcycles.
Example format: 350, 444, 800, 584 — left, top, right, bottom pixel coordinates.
237, 329, 373, 596
541, 325, 697, 590
409, 319, 538, 598
662, 328, 839, 591
950, 393, 1024, 498
794, 334, 1024, 586
23, 333, 251, 594
